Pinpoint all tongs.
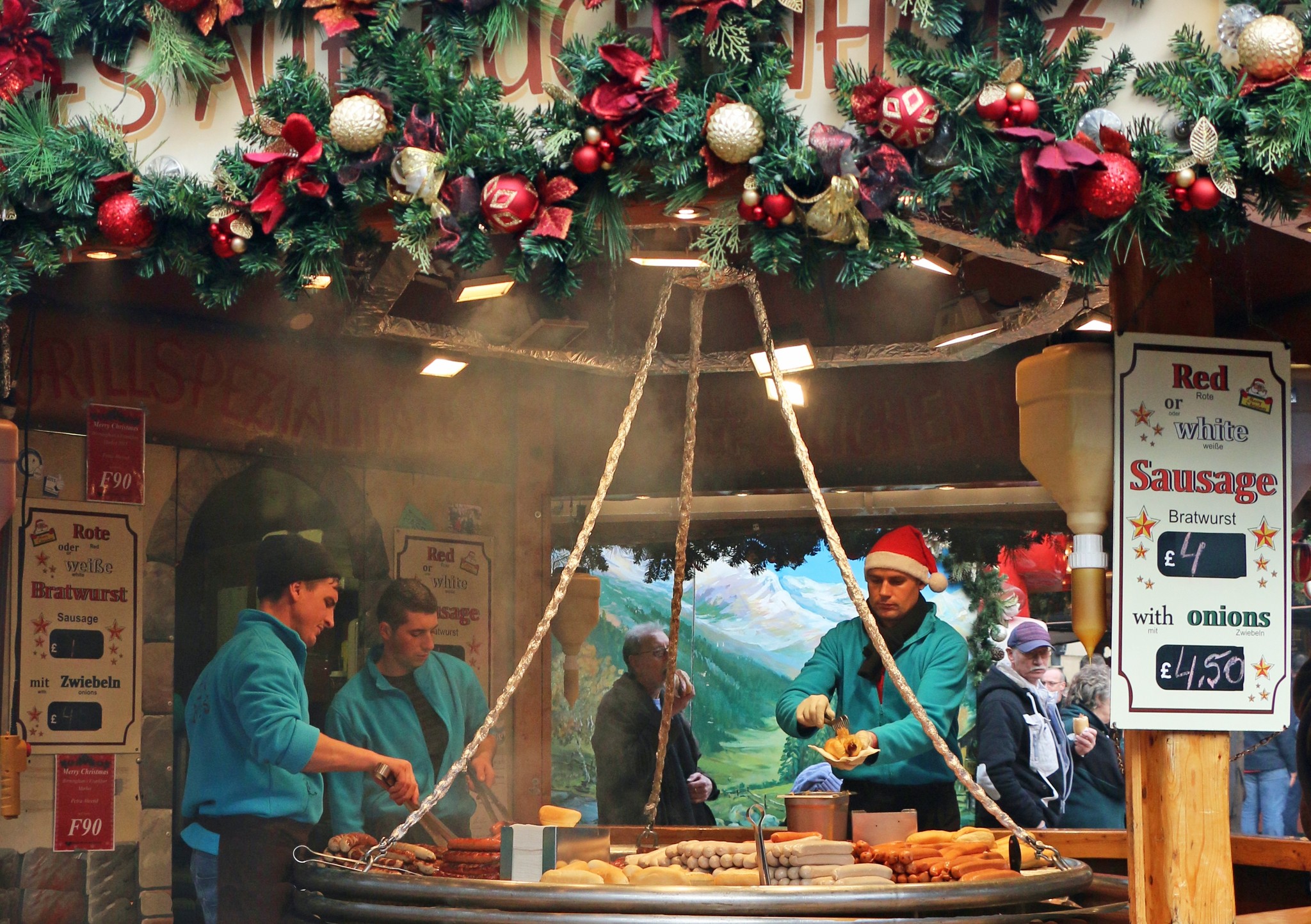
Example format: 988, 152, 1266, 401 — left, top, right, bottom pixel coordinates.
376, 762, 460, 845
746, 803, 771, 886
292, 845, 428, 878
824, 712, 849, 731
466, 761, 513, 824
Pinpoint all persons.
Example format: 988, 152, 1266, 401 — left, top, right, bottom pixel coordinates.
1059, 663, 1126, 830
181, 534, 420, 924
775, 524, 970, 832
1229, 654, 1311, 842
591, 621, 721, 826
975, 621, 1099, 829
1040, 667, 1067, 711
1079, 653, 1105, 669
323, 575, 498, 849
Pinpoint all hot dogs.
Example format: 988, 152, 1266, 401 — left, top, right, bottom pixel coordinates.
674, 669, 691, 697
824, 728, 864, 758
1073, 715, 1088, 736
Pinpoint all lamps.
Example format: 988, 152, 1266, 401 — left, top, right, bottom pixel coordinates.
749, 323, 817, 380
417, 347, 469, 379
1071, 310, 1113, 331
764, 375, 812, 408
625, 226, 715, 267
445, 248, 515, 304
899, 244, 963, 277
59, 238, 132, 266
504, 294, 590, 352
1039, 227, 1087, 268
300, 271, 332, 290
926, 295, 1004, 348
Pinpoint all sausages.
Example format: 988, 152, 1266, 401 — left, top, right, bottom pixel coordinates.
853, 839, 1027, 883
318, 820, 514, 880
541, 832, 896, 885
908, 825, 1053, 867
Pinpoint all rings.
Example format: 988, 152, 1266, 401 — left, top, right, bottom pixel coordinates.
695, 788, 698, 793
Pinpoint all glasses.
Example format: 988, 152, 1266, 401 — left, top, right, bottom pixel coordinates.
632, 645, 670, 658
1014, 647, 1053, 660
1041, 681, 1061, 687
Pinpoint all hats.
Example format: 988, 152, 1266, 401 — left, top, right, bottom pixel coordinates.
255, 534, 342, 590
1008, 621, 1056, 653
790, 762, 843, 793
864, 524, 948, 592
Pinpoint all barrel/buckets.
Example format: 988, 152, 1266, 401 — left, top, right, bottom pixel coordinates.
775, 790, 856, 841
